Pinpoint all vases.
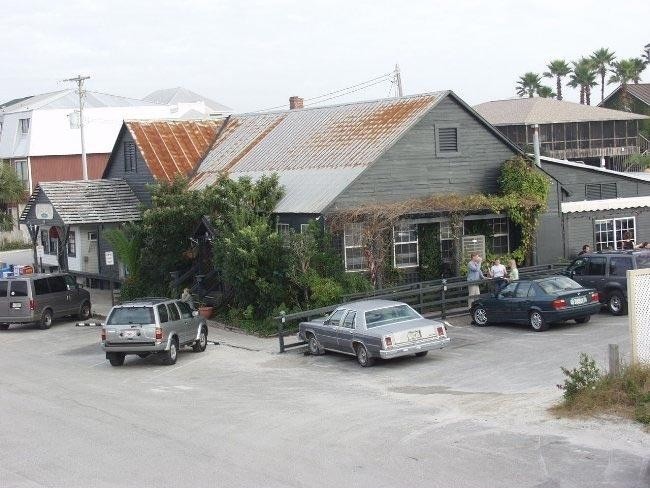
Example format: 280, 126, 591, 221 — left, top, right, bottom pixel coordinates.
199, 305, 214, 319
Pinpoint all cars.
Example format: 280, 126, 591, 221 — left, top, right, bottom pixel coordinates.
469, 273, 597, 332
293, 298, 451, 364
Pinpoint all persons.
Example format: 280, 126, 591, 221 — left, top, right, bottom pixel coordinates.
487, 257, 508, 290
577, 244, 589, 255
180, 287, 195, 310
507, 258, 519, 281
465, 253, 487, 308
621, 231, 642, 249
642, 242, 650, 249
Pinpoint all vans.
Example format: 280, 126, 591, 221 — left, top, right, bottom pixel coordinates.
0, 272, 92, 331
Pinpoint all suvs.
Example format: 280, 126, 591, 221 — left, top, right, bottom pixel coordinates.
562, 249, 650, 317
101, 293, 208, 367
